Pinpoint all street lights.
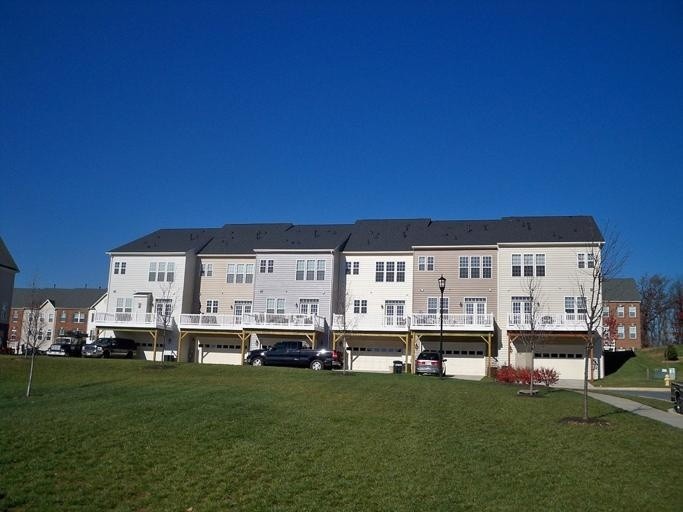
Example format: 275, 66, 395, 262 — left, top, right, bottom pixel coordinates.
436, 273, 446, 376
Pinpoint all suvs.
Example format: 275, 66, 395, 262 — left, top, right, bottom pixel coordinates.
81, 337, 138, 360
45, 331, 87, 357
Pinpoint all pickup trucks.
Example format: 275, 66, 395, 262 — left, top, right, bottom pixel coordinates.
243, 339, 342, 371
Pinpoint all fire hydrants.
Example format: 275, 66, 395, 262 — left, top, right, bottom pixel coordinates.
663, 374, 670, 386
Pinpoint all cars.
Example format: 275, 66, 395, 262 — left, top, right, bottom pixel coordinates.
413, 351, 447, 376
